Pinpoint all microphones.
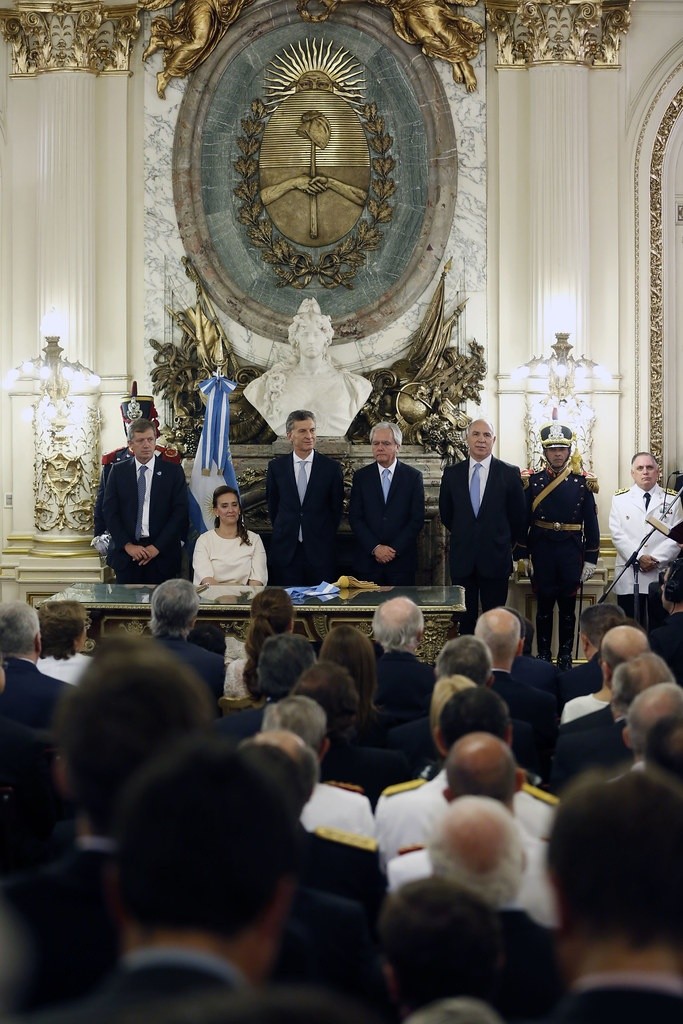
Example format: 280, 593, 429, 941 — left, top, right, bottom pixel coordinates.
671, 470, 683, 474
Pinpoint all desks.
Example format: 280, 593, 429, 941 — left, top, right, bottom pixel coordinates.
32, 580, 472, 663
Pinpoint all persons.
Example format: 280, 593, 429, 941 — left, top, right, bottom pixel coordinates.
523, 424, 599, 666
192, 486, 268, 590
91, 394, 189, 587
609, 453, 683, 625
348, 422, 431, 587
0, 579, 683, 1024
439, 420, 528, 635
197, 586, 265, 605
265, 409, 343, 585
93, 583, 160, 603
243, 298, 373, 437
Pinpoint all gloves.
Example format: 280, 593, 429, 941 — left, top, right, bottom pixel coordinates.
91, 536, 107, 556
520, 559, 534, 576
580, 562, 598, 583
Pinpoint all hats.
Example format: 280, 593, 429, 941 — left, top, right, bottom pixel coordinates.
538, 422, 577, 447
120, 395, 155, 423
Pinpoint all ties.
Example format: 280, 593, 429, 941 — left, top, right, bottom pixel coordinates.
382, 469, 390, 503
131, 467, 147, 541
295, 461, 308, 542
644, 493, 651, 511
470, 463, 481, 517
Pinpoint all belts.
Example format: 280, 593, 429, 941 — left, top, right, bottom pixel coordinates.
535, 520, 581, 531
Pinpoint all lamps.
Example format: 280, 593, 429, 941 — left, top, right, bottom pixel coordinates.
506, 296, 619, 422
4, 306, 112, 426
